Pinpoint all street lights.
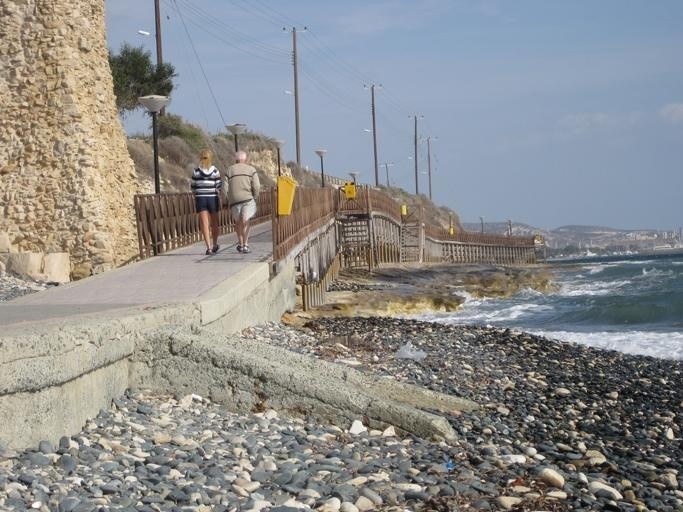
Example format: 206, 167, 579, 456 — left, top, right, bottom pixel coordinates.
136, 30, 165, 116
270, 140, 286, 177
315, 149, 327, 188
284, 89, 301, 170
348, 171, 359, 187
225, 122, 247, 164
379, 159, 396, 188
360, 128, 379, 188
137, 93, 171, 195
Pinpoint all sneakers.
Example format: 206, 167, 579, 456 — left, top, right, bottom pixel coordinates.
236, 245, 251, 253
206, 245, 220, 255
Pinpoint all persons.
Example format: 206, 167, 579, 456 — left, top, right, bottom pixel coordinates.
221, 150, 259, 253
189, 149, 222, 256
